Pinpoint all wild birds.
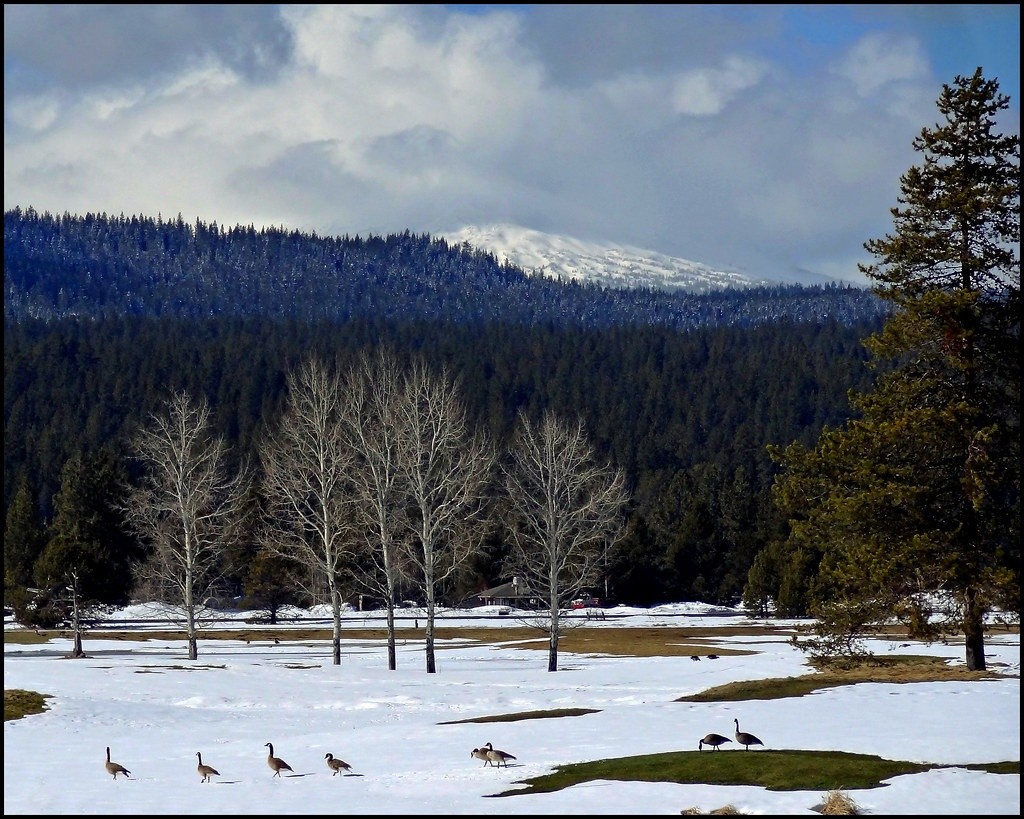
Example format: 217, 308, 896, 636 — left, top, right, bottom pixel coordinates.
691, 653, 701, 662
34, 629, 41, 636
194, 752, 220, 783
105, 746, 132, 781
734, 718, 764, 751
59, 630, 67, 638
706, 654, 720, 659
698, 733, 733, 751
470, 742, 517, 769
264, 742, 294, 778
323, 752, 353, 777
